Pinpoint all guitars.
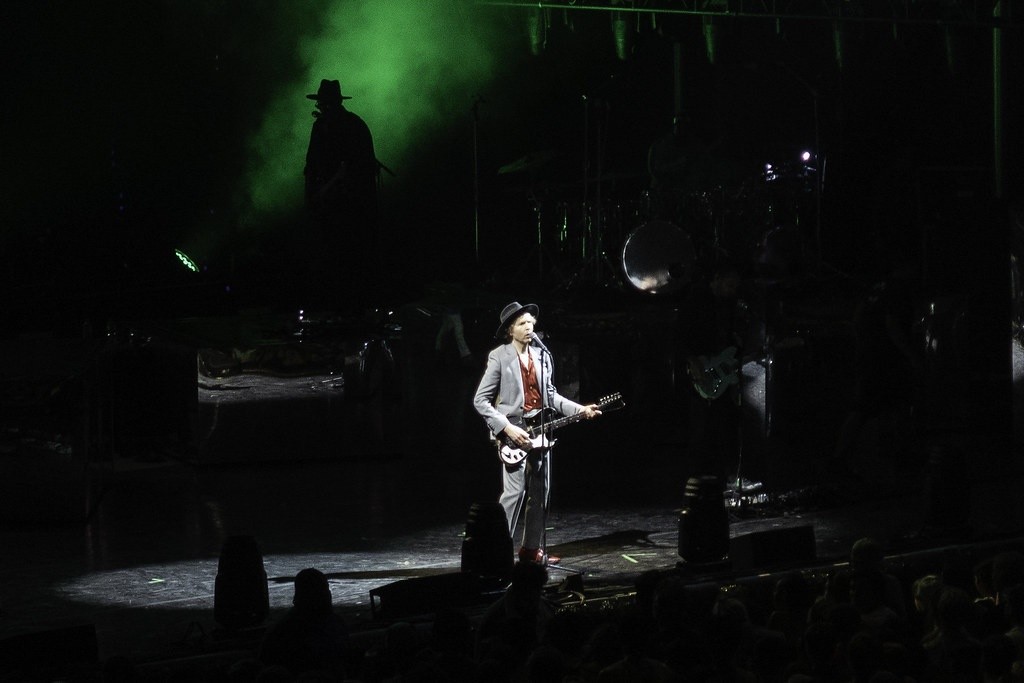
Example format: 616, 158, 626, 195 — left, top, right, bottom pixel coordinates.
496, 392, 626, 466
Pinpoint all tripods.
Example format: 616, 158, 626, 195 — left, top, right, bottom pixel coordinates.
538, 345, 585, 574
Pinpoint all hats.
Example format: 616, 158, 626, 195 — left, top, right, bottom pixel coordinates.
305, 79, 352, 101
492, 302, 540, 343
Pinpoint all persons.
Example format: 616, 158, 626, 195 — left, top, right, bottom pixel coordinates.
223, 537, 1024, 683
472, 300, 602, 566
298, 80, 383, 217
664, 264, 768, 490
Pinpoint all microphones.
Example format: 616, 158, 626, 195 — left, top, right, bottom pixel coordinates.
529, 332, 552, 355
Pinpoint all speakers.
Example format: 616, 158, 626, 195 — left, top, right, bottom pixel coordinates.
730, 525, 816, 569
370, 574, 480, 619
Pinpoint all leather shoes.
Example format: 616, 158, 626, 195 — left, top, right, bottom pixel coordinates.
518, 546, 561, 564
